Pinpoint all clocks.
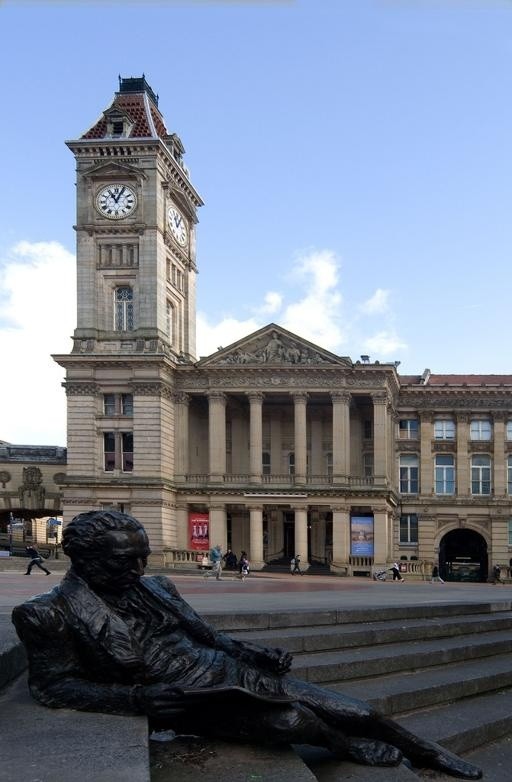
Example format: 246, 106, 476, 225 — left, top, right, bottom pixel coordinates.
92, 183, 138, 221
165, 205, 190, 247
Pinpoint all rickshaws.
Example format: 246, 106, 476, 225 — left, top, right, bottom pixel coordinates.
373, 568, 391, 582
196, 559, 227, 570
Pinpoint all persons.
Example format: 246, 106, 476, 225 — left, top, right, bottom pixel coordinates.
12, 508, 485, 779
491, 564, 504, 584
262, 331, 284, 364
239, 549, 250, 574
222, 549, 237, 570
429, 563, 444, 584
241, 557, 248, 582
290, 554, 304, 575
286, 341, 301, 365
24, 542, 54, 576
388, 563, 404, 582
205, 542, 224, 581
300, 348, 311, 365
